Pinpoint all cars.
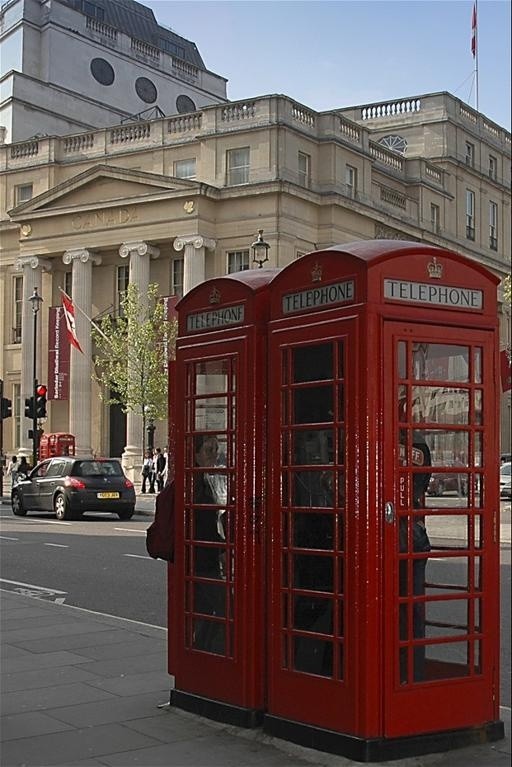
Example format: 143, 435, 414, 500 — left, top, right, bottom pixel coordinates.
10, 454, 137, 520
421, 450, 511, 501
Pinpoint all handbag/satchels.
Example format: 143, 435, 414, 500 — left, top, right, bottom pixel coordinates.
146, 480, 177, 560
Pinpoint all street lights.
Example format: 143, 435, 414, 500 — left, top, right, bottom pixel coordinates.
143, 404, 158, 486
250, 225, 270, 270
26, 287, 47, 473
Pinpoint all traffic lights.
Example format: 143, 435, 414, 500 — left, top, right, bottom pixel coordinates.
23, 396, 34, 419
36, 385, 47, 418
2, 398, 13, 419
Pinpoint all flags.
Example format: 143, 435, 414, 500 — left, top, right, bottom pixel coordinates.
470, 2, 478, 58
62, 292, 85, 354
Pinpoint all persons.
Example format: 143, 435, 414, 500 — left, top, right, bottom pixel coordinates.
17, 457, 31, 480
394, 397, 432, 682
6, 455, 21, 488
140, 445, 168, 494
190, 429, 222, 653
